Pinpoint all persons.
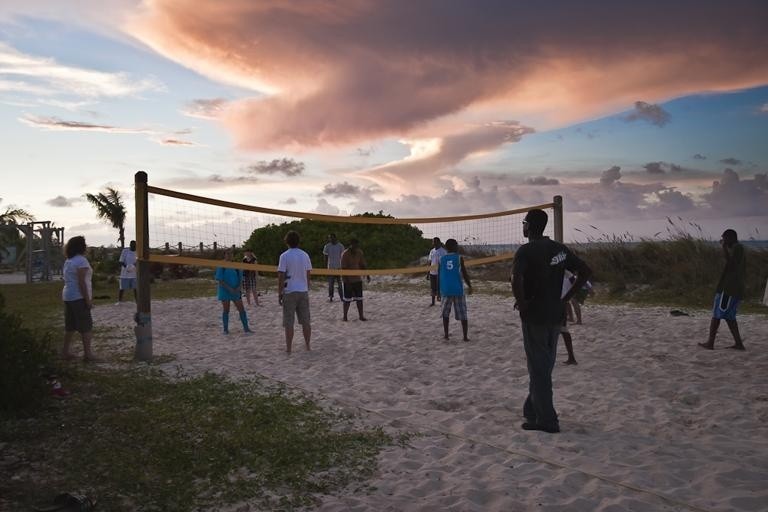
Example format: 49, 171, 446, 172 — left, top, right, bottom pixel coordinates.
567, 274, 595, 325
509, 209, 592, 433
62, 236, 99, 360
214, 230, 371, 354
115, 240, 137, 304
698, 229, 746, 350
560, 269, 578, 364
435, 238, 473, 341
426, 237, 446, 306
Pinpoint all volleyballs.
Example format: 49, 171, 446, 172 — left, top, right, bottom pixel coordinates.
126, 264, 136, 272
